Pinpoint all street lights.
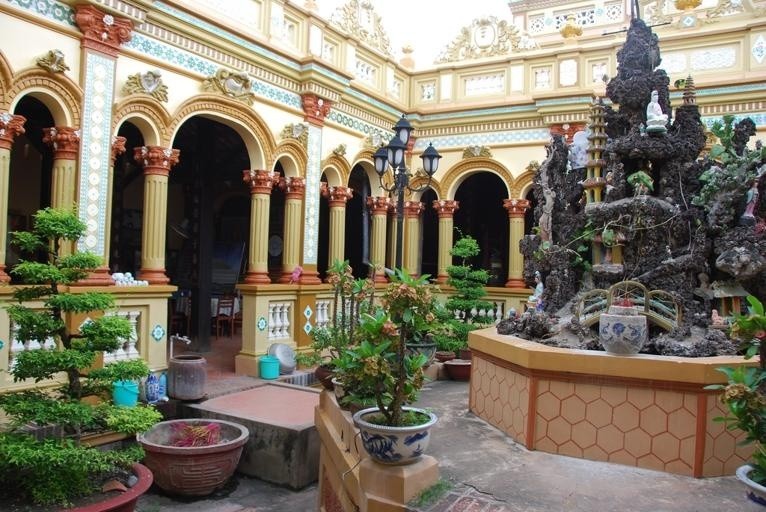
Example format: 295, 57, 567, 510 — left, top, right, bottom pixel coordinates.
371, 112, 442, 279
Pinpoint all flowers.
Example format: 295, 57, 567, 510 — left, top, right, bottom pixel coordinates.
170, 421, 221, 448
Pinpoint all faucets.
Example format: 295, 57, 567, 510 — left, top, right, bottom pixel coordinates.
168, 333, 192, 357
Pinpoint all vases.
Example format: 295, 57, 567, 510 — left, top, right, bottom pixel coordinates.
138, 418, 250, 498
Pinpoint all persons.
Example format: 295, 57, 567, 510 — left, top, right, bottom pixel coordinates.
744, 178, 759, 217
645, 90, 668, 124
527, 271, 544, 302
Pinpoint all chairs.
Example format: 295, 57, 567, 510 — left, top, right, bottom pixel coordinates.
212, 295, 236, 339
169, 300, 186, 336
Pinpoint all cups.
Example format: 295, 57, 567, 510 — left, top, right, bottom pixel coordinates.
116, 277, 148, 288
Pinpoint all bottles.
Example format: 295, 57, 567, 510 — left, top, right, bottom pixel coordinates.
146, 369, 167, 403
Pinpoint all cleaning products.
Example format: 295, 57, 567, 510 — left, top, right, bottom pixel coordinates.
159, 372, 168, 399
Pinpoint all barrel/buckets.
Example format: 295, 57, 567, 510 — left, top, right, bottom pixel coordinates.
259, 349, 280, 380
169, 355, 207, 400
112, 380, 141, 407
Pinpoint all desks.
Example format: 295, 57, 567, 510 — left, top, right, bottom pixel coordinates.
211, 298, 240, 318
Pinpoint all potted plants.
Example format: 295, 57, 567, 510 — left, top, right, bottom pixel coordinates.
294, 235, 496, 412
335, 269, 454, 466
703, 296, 766, 512
0, 209, 163, 512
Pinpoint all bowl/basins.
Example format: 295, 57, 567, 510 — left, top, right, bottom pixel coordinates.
267, 340, 297, 375
